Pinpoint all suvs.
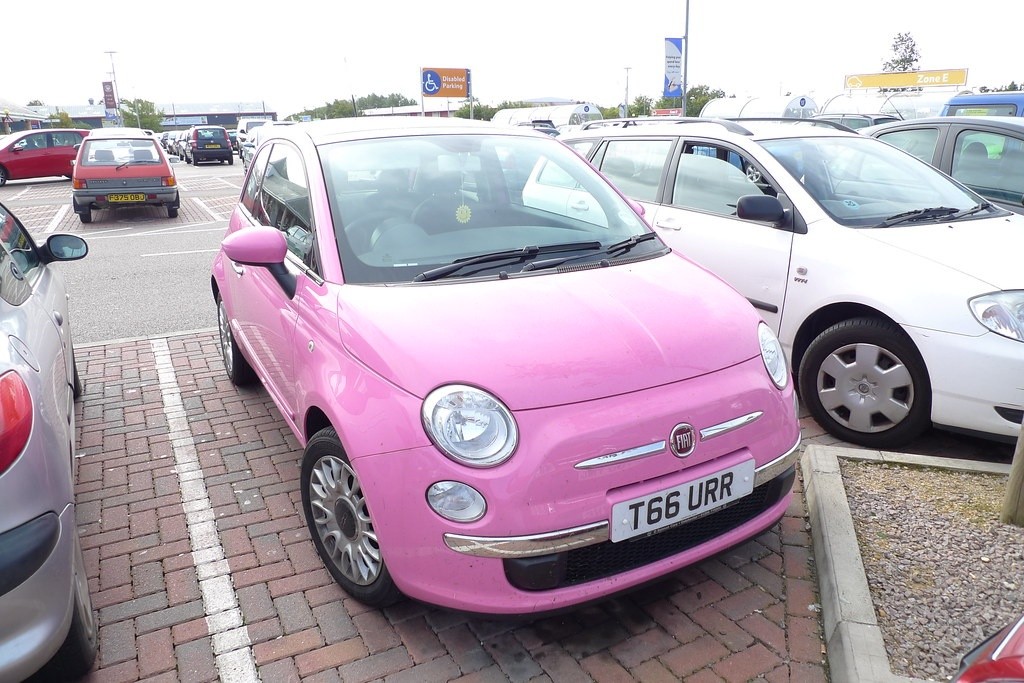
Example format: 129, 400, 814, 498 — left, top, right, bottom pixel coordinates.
520, 115, 1024, 451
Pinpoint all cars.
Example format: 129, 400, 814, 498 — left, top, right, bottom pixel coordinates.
0, 127, 92, 186
791, 114, 903, 136
71, 126, 180, 224
209, 112, 809, 624
0, 201, 100, 683
503, 118, 561, 138
800, 116, 1024, 219
153, 118, 299, 174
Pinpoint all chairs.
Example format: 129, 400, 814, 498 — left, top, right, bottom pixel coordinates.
289, 168, 497, 256
953, 142, 992, 187
95, 149, 154, 163
618, 152, 819, 219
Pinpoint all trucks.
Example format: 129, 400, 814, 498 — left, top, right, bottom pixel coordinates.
936, 93, 1024, 171
